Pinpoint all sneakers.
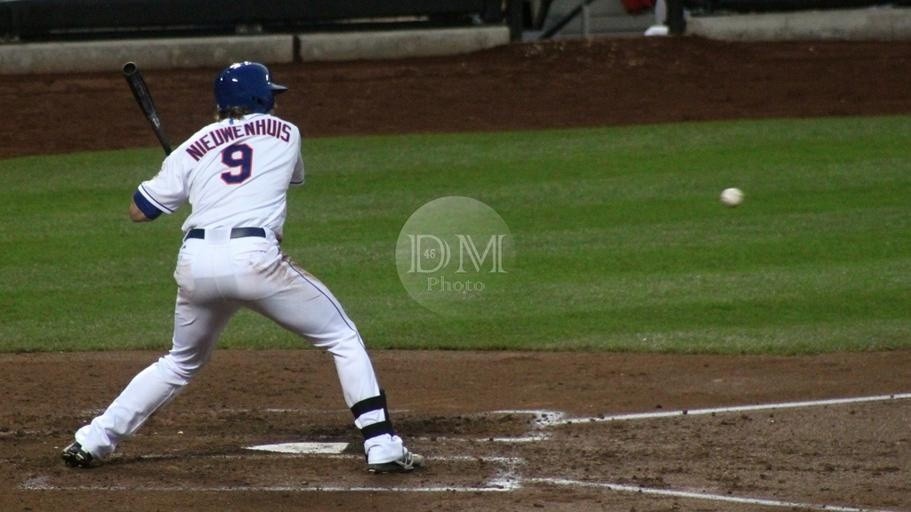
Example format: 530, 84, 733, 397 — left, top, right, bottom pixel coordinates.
368, 451, 426, 474
60, 441, 94, 468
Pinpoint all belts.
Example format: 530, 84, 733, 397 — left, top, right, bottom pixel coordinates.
184, 227, 266, 242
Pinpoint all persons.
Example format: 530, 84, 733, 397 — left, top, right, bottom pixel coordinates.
60, 61, 425, 474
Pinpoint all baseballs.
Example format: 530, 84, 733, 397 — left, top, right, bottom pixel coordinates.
720, 187, 743, 205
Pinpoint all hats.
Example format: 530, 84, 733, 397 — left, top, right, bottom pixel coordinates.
214, 61, 289, 112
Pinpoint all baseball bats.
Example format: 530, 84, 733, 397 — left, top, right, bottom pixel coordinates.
121, 62, 173, 156
